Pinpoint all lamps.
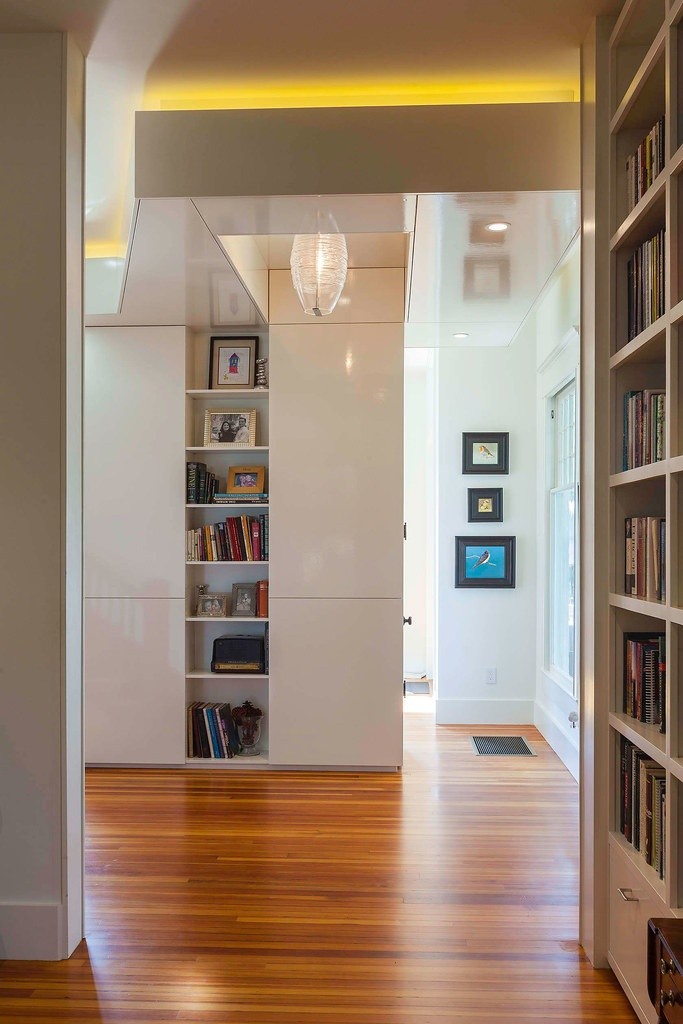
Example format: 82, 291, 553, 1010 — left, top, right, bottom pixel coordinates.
289, 234, 347, 316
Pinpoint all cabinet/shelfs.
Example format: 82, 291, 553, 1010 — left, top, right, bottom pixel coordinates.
182, 323, 270, 770
580, 0, 682, 1024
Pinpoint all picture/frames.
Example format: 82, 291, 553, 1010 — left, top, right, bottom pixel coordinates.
465, 253, 512, 302
461, 431, 510, 475
204, 408, 256, 447
226, 466, 265, 494
196, 593, 226, 617
230, 582, 258, 615
208, 333, 256, 388
455, 534, 516, 589
467, 487, 504, 522
208, 263, 258, 327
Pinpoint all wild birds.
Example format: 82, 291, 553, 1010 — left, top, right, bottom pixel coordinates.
478, 445, 494, 460
473, 550, 491, 570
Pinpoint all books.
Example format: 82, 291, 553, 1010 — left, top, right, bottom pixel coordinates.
619, 734, 667, 883
621, 632, 666, 736
188, 460, 219, 505
186, 513, 269, 561
623, 390, 665, 472
623, 119, 664, 218
212, 493, 269, 504
623, 517, 666, 603
185, 701, 240, 760
258, 580, 268, 618
626, 230, 666, 343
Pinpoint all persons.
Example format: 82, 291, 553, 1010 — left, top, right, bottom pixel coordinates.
204, 599, 223, 613
238, 475, 255, 487
234, 417, 249, 443
219, 422, 234, 442
